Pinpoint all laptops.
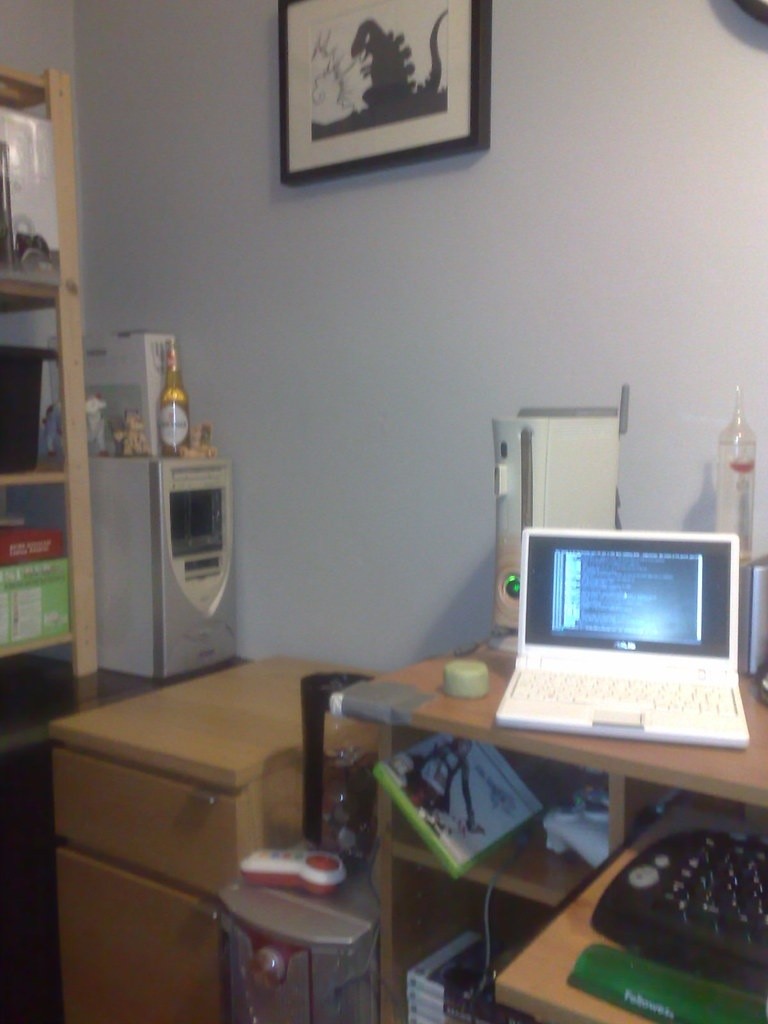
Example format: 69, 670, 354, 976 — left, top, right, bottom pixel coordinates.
495, 527, 750, 751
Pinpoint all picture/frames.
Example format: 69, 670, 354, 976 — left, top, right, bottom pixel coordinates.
280, 2, 492, 182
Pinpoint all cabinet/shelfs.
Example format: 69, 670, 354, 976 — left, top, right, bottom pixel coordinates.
0, 69, 98, 752
46, 653, 388, 1023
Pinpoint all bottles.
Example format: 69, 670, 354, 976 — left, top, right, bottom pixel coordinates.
717, 383, 754, 562
161, 338, 191, 456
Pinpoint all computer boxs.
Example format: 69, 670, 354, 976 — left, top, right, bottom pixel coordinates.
15, 452, 238, 678
217, 862, 382, 1024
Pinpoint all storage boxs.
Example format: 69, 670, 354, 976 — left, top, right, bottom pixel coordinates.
0, 108, 61, 283
1, 528, 70, 646
39, 332, 176, 462
1, 345, 56, 472
407, 936, 521, 1023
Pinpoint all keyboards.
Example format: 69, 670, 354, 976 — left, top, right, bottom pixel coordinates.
590, 828, 768, 998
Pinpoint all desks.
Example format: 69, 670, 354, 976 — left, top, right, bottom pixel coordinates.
329, 631, 768, 1023
